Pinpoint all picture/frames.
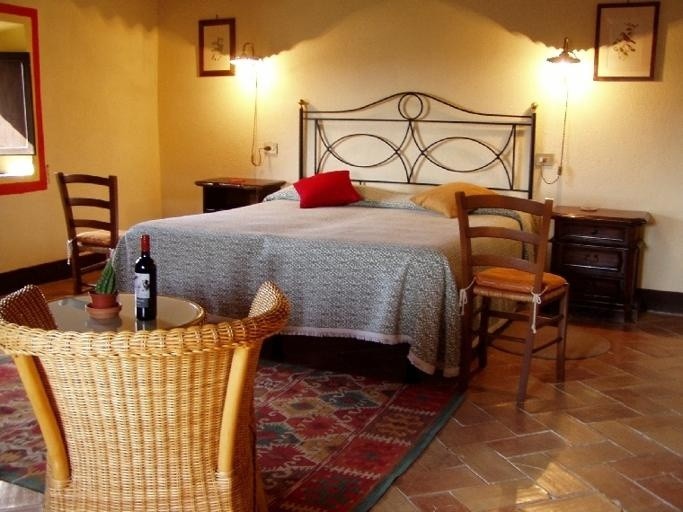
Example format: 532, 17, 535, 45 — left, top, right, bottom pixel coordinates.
593, 1, 658, 84
198, 18, 236, 77
0, 51, 36, 157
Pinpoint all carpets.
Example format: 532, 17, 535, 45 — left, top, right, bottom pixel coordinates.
1, 293, 469, 511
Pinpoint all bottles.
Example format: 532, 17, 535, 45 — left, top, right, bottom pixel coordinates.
133, 234, 157, 322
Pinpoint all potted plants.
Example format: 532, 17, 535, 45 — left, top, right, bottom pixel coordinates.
85, 261, 126, 324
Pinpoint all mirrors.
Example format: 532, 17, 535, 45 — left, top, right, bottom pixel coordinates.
0, 3, 48, 196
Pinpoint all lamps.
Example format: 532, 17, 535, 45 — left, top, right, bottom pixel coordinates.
537, 36, 582, 184
229, 42, 277, 167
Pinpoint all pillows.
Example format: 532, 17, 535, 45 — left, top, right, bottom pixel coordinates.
409, 179, 504, 219
292, 170, 365, 209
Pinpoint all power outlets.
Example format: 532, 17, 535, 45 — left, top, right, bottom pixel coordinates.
535, 153, 554, 167
262, 143, 278, 154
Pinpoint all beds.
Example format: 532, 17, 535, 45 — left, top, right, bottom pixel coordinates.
96, 91, 537, 382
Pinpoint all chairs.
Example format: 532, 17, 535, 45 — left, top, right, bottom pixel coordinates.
54, 171, 126, 296
455, 190, 569, 402
0, 280, 291, 512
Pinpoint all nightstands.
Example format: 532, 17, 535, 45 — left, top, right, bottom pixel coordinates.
194, 177, 287, 213
547, 200, 652, 324
